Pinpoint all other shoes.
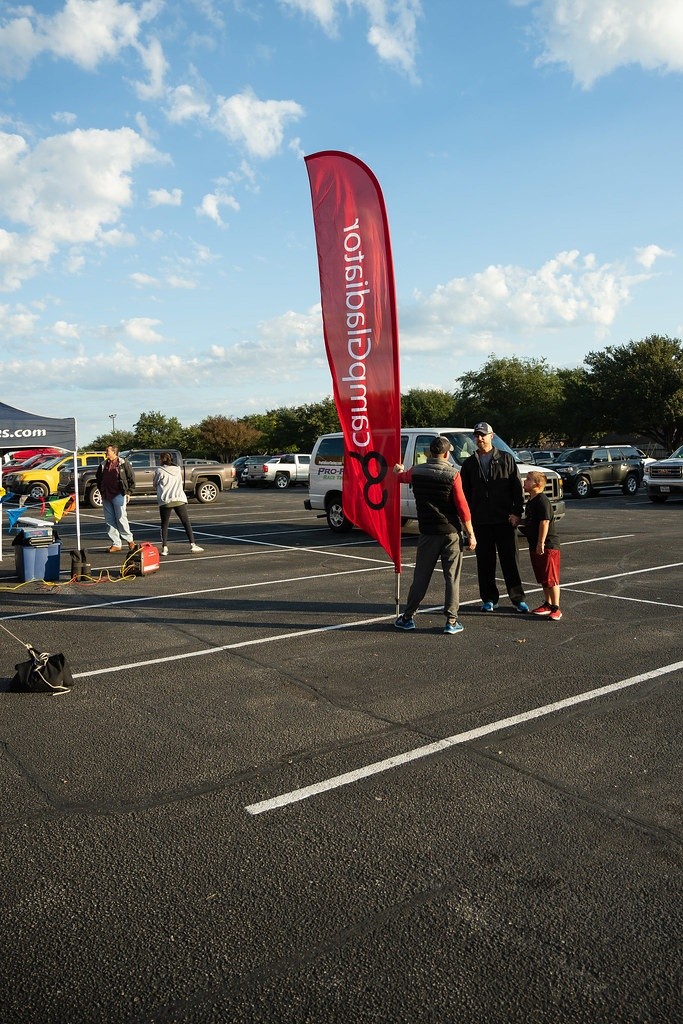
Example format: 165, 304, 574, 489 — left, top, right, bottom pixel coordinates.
190, 546, 204, 553
106, 545, 121, 552
129, 540, 136, 550
160, 550, 169, 556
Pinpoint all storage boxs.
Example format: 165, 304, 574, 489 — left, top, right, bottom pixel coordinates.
15, 539, 63, 583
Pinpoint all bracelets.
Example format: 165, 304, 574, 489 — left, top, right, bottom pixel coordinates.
396, 468, 403, 473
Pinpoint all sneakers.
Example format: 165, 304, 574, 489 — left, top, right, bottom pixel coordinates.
393, 615, 416, 630
481, 601, 499, 612
532, 600, 552, 615
513, 601, 529, 612
444, 621, 464, 634
546, 605, 563, 620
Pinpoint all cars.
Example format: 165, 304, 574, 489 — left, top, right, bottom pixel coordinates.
637, 449, 657, 485
508, 448, 564, 466
2, 457, 27, 467
229, 455, 272, 477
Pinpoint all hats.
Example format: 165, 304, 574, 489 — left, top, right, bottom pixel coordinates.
473, 422, 492, 434
430, 436, 455, 453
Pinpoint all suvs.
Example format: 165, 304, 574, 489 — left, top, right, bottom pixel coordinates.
302, 427, 566, 545
643, 445, 683, 503
539, 444, 646, 499
1, 452, 60, 478
1, 451, 106, 503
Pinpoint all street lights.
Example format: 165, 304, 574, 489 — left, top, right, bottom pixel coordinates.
109, 413, 117, 434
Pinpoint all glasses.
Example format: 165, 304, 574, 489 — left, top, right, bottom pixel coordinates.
475, 433, 490, 437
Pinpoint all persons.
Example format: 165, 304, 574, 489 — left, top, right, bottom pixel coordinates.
393, 437, 477, 633
95, 444, 136, 553
459, 422, 530, 612
510, 470, 564, 620
152, 453, 203, 555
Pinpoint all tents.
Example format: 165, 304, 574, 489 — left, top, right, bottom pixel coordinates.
0, 402, 81, 551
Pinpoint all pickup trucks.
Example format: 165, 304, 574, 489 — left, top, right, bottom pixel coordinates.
242, 453, 312, 489
57, 449, 237, 508
